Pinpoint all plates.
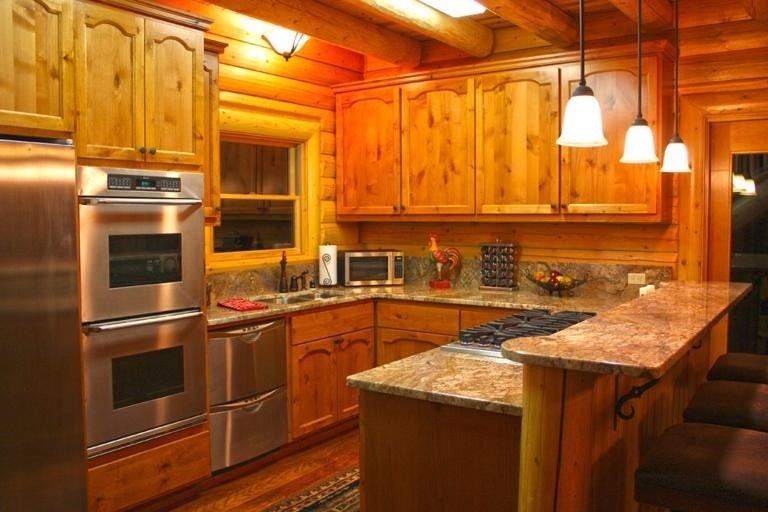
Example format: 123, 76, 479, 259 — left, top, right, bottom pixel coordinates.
527, 274, 587, 296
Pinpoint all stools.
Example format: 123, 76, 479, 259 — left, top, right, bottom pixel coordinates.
705, 352, 768, 380
682, 380, 768, 431
634, 421, 768, 511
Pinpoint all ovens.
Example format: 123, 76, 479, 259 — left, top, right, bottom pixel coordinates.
77, 166, 212, 512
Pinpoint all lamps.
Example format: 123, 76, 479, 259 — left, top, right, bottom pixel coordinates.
619, 1, 661, 163
659, 1, 693, 173
556, 0, 610, 149
739, 179, 756, 196
733, 174, 747, 192
261, 25, 311, 60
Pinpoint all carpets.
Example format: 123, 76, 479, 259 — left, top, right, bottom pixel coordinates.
260, 463, 361, 511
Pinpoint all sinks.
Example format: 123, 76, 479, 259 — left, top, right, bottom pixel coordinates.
299, 292, 342, 300
255, 297, 309, 308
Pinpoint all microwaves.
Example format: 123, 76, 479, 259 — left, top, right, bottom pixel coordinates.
344, 249, 404, 286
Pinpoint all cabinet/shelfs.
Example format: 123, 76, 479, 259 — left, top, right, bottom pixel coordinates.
475, 56, 663, 215
375, 299, 458, 370
334, 75, 475, 215
1, 1, 77, 133
79, 0, 212, 166
289, 301, 374, 441
462, 305, 550, 338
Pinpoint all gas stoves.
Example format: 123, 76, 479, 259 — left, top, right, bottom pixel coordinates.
441, 309, 594, 362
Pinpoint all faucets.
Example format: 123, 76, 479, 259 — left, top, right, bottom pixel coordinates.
291, 273, 304, 290
